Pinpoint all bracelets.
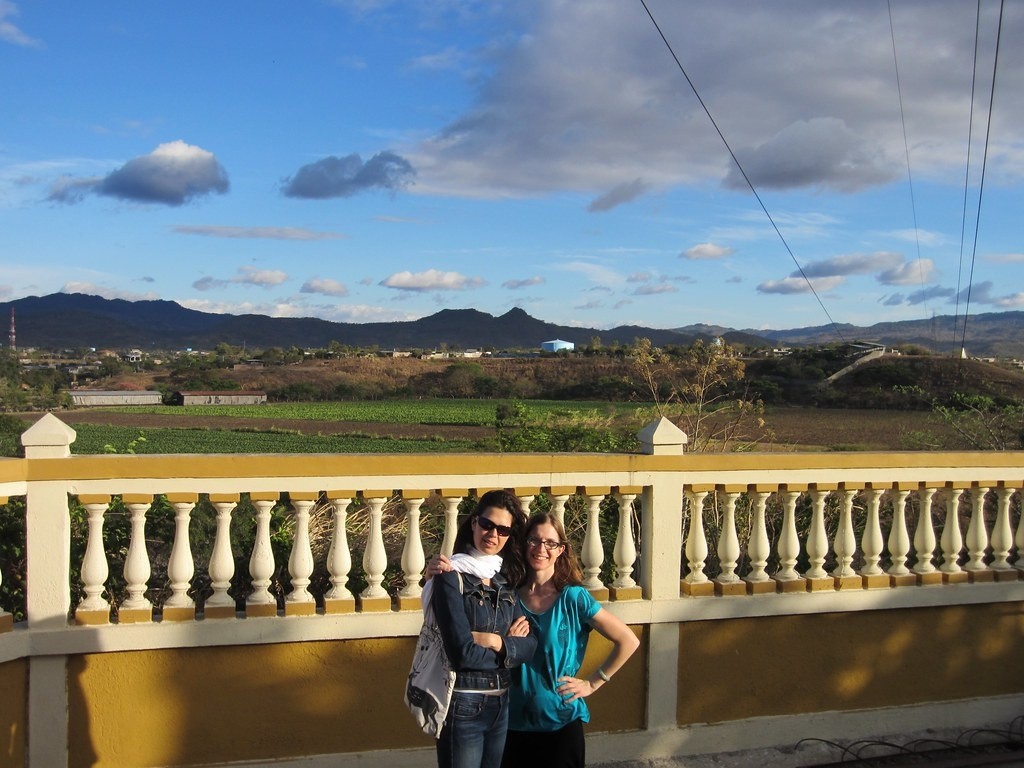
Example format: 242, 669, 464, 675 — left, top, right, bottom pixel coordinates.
597, 667, 610, 682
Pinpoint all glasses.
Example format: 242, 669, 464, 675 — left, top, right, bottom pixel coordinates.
526, 537, 560, 550
476, 516, 512, 537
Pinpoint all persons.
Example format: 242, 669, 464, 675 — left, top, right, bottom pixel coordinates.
420, 511, 640, 768
429, 489, 539, 768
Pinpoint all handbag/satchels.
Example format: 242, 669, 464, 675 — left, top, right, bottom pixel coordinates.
404, 571, 464, 739
